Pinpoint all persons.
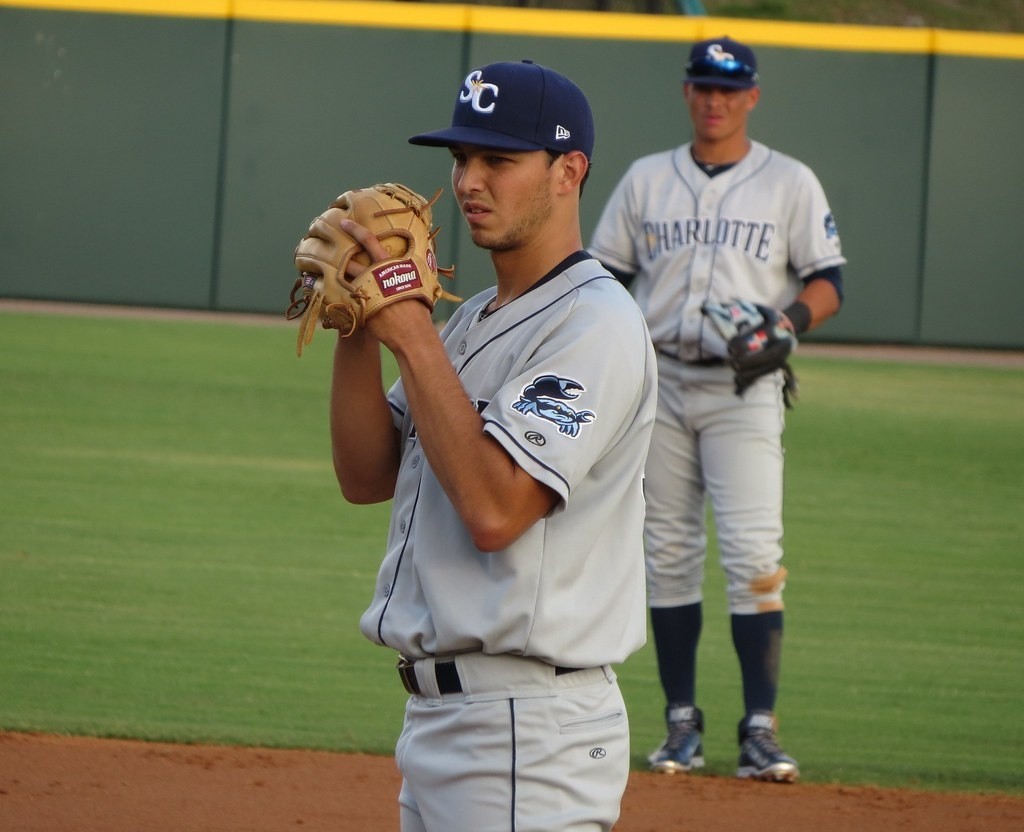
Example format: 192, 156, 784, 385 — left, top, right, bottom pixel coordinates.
287, 58, 659, 832
586, 35, 847, 782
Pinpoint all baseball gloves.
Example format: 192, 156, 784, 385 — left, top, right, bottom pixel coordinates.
269, 181, 468, 358
727, 302, 800, 412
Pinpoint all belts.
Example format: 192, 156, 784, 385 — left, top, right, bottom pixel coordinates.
400, 651, 580, 695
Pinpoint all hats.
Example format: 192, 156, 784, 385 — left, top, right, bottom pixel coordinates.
681, 36, 757, 89
408, 58, 595, 163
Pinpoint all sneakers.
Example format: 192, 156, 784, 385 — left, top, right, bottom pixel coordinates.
735, 708, 800, 782
648, 701, 705, 775
